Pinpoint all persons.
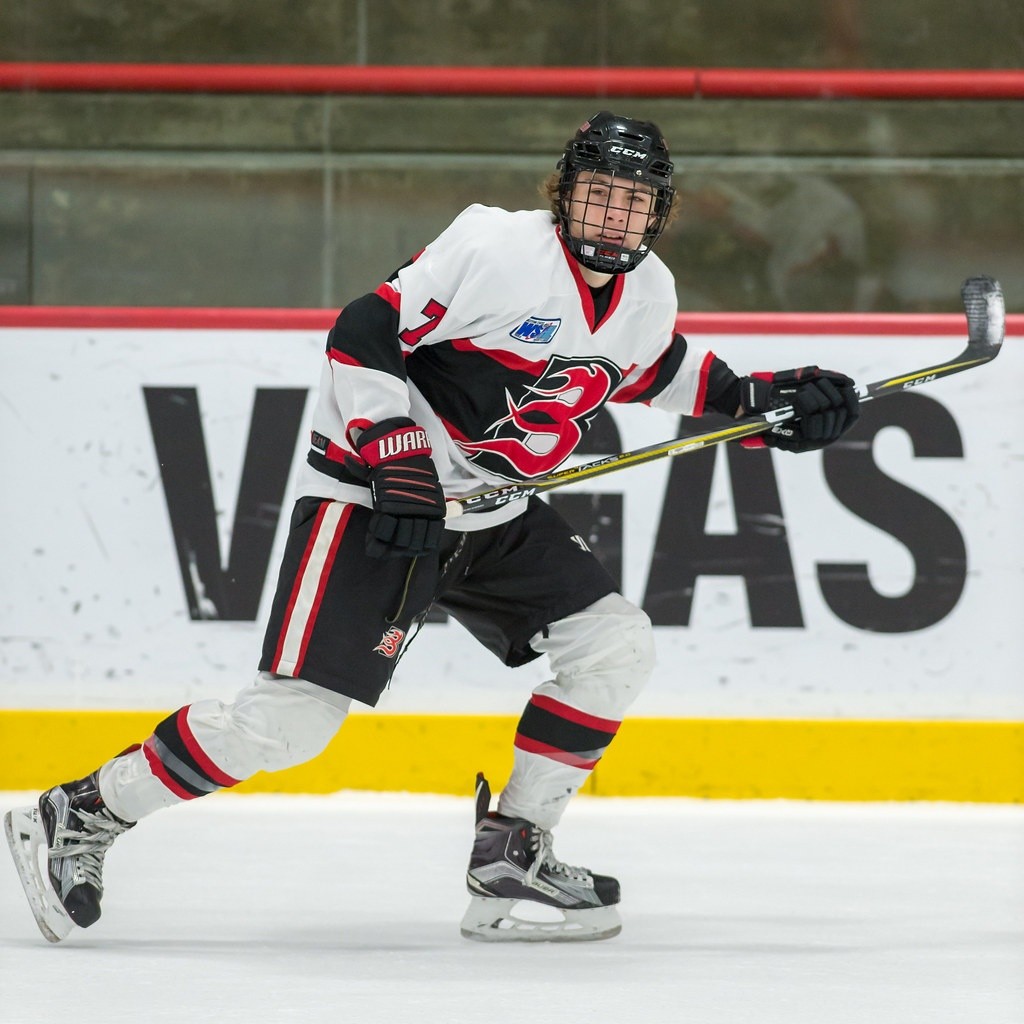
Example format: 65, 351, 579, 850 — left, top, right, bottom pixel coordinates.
4, 111, 857, 944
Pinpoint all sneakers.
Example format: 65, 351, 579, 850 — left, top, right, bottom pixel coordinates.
5, 742, 140, 942
459, 776, 628, 937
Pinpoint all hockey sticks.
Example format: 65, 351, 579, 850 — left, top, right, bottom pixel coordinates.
446, 274, 1005, 521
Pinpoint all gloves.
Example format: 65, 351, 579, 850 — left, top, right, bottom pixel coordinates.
734, 365, 856, 449
355, 417, 449, 559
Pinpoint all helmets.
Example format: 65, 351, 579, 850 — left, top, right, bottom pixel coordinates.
551, 113, 671, 277
